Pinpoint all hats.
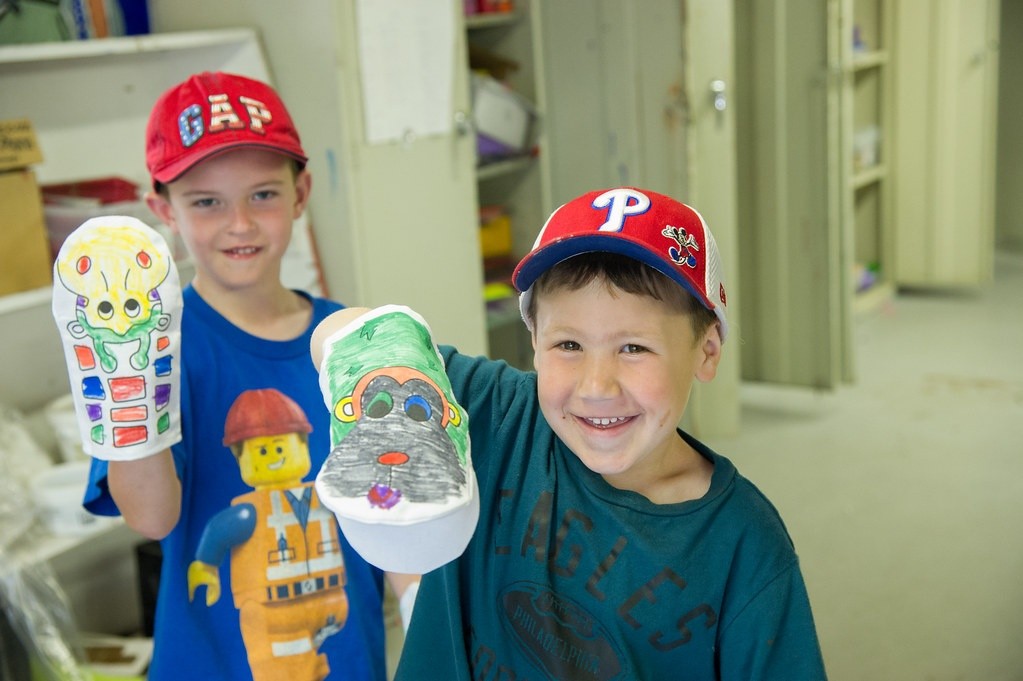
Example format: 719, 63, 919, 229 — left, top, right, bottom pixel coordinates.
144, 69, 315, 187
511, 186, 736, 344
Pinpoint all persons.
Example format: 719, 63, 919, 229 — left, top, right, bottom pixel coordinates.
305, 182, 832, 681
60, 69, 427, 681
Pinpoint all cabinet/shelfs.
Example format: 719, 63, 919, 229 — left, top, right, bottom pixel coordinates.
2, 26, 326, 680
733, 2, 1003, 392
345, 2, 552, 374
